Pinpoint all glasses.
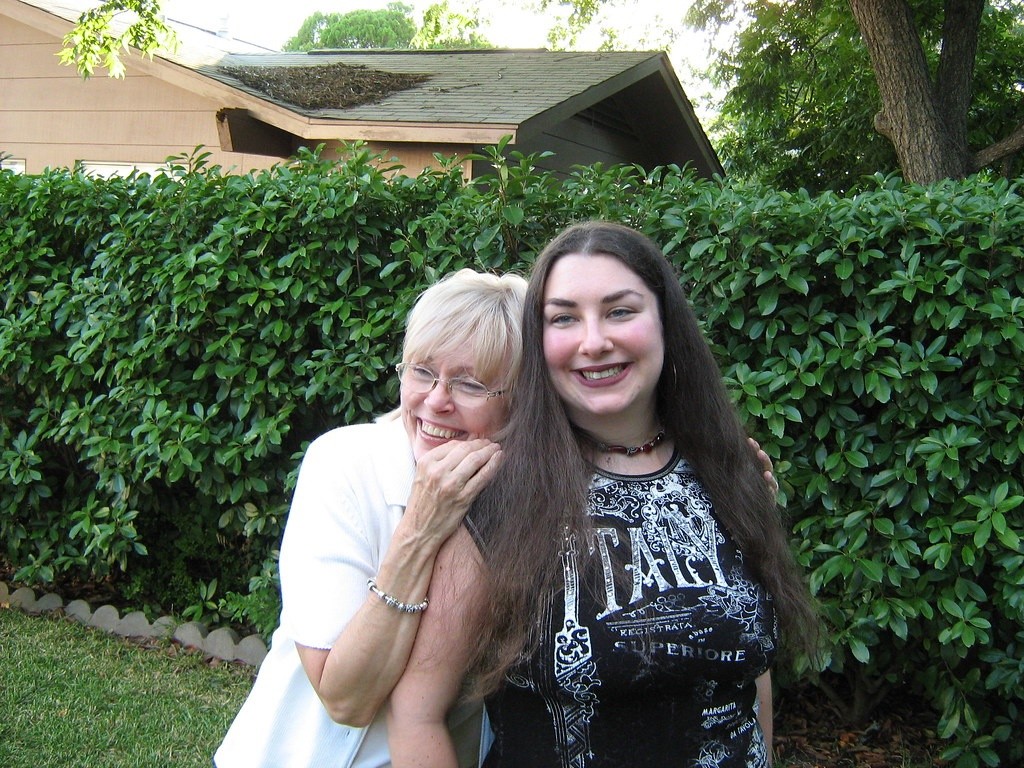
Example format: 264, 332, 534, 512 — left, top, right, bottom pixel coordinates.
395, 361, 508, 408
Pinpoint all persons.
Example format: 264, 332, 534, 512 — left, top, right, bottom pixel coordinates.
212, 270, 780, 768
384, 220, 820, 768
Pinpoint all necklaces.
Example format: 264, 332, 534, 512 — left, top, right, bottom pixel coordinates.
571, 426, 668, 456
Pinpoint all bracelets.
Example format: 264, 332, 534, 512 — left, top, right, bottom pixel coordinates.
368, 579, 428, 612
